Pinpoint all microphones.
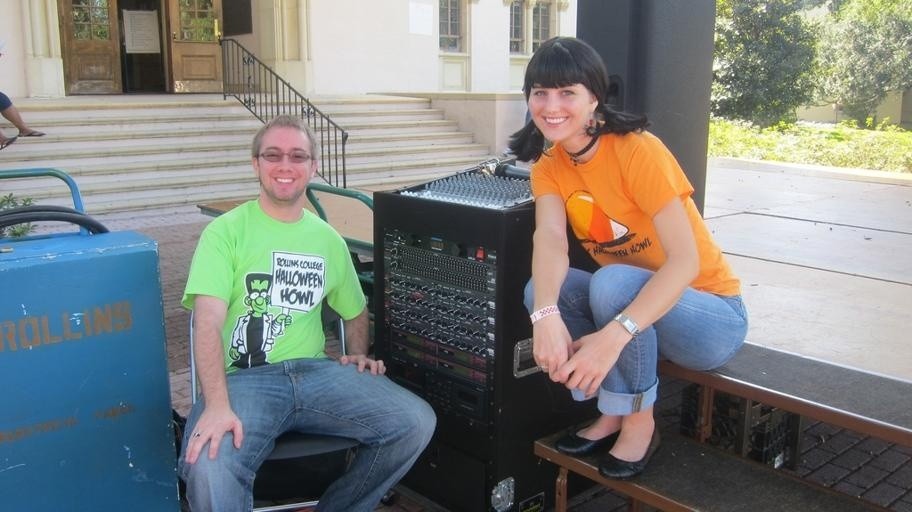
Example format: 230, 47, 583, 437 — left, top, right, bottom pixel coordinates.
494, 164, 533, 181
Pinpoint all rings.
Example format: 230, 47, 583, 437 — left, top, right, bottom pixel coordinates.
193, 432, 200, 439
541, 365, 548, 370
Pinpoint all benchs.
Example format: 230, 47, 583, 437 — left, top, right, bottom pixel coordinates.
657, 340, 912, 471
534, 418, 891, 511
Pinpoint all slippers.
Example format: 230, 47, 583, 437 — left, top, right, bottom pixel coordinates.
0, 137, 17, 150
18, 131, 46, 136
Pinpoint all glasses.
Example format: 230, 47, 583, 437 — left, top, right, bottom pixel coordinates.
256, 150, 313, 163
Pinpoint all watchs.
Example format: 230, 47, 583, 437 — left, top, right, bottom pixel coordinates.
615, 313, 639, 335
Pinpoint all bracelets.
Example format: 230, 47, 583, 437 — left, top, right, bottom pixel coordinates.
530, 305, 561, 325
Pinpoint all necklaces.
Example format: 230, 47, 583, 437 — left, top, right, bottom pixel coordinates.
568, 121, 600, 166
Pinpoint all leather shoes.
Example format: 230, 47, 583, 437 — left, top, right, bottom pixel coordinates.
554, 425, 620, 456
599, 422, 661, 480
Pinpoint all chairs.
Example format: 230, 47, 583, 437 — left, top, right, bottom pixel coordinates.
188, 291, 370, 511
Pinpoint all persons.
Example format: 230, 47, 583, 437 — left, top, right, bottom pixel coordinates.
177, 112, 439, 511
509, 35, 747, 479
0, 92, 46, 149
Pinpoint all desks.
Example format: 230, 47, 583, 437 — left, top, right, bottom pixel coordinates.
197, 185, 377, 279
